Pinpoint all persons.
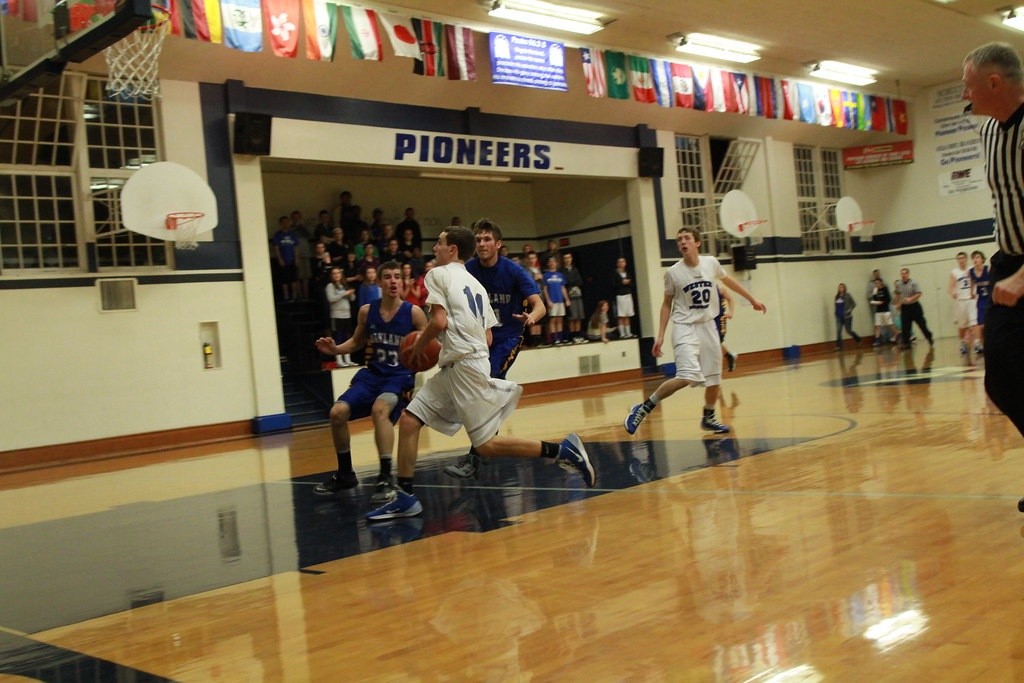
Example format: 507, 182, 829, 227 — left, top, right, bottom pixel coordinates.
272, 191, 479, 368
833, 283, 863, 350
366, 227, 596, 518
950, 251, 990, 366
587, 300, 616, 343
866, 267, 934, 348
713, 288, 737, 372
502, 242, 588, 348
625, 227, 767, 434
962, 43, 1024, 512
316, 261, 428, 500
614, 259, 638, 338
444, 218, 546, 478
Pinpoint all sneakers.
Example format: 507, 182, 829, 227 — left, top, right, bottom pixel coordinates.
313, 471, 362, 497
619, 333, 627, 339
624, 403, 651, 435
442, 454, 479, 478
373, 473, 391, 499
551, 432, 595, 487
365, 484, 423, 520
335, 360, 348, 368
701, 407, 729, 436
345, 359, 359, 367
626, 333, 634, 339
727, 353, 738, 371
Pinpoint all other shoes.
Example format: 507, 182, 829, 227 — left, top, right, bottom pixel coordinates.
899, 342, 912, 350
960, 344, 968, 353
835, 344, 842, 351
872, 338, 881, 346
925, 335, 934, 345
974, 346, 984, 353
532, 337, 590, 348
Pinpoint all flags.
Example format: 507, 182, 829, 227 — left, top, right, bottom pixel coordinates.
580, 47, 908, 134
0, 0, 476, 84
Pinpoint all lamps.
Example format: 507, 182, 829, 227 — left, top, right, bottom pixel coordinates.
487, 0, 605, 36
1001, 8, 1024, 32
809, 60, 878, 86
675, 34, 761, 64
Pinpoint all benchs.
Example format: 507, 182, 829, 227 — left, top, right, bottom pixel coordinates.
271, 254, 641, 374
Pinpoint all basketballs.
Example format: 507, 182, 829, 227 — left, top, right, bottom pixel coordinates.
399, 332, 440, 371
